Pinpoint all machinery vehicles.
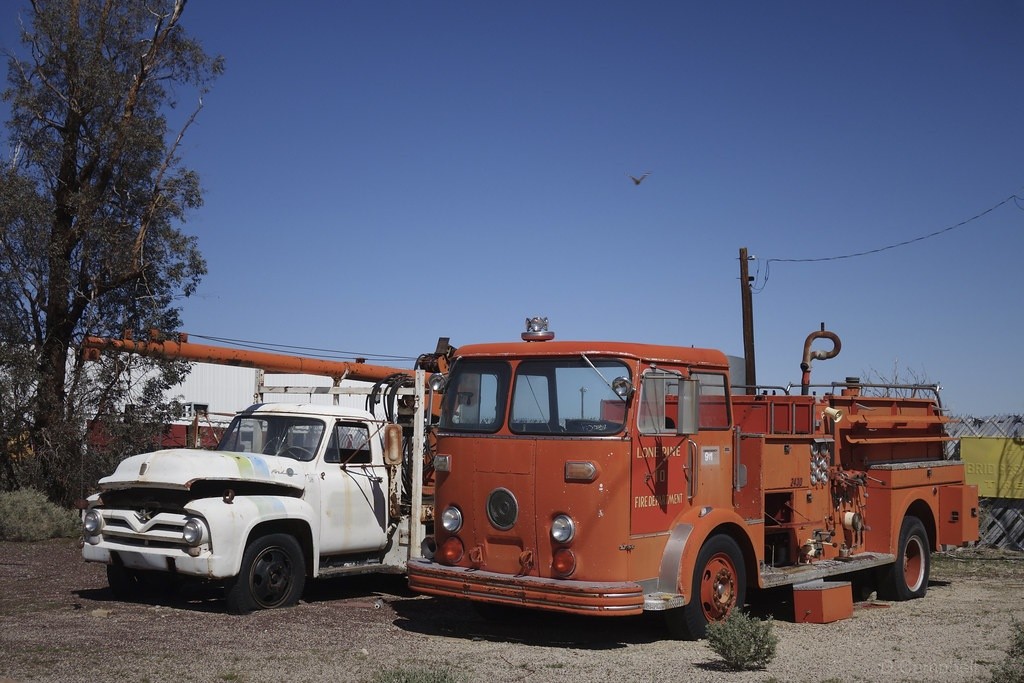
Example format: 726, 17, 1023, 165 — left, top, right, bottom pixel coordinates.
74, 328, 458, 613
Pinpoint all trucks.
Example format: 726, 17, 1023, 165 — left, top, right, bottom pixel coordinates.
405, 316, 979, 644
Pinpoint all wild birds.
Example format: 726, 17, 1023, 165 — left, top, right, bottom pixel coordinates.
627, 171, 651, 185
